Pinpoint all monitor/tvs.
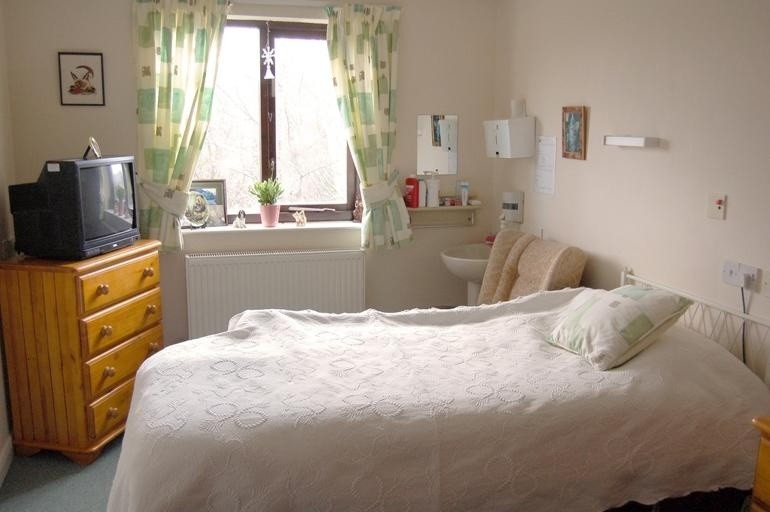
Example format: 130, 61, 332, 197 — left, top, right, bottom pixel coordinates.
8, 153, 141, 259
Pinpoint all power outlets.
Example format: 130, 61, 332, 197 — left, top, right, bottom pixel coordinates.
738, 263, 763, 295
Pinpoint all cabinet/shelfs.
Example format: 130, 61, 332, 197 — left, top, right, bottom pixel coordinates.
1, 234, 166, 474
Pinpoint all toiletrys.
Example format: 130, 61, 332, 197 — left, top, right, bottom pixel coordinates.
417, 178, 428, 207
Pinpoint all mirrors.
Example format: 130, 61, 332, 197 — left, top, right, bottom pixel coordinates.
416, 112, 458, 176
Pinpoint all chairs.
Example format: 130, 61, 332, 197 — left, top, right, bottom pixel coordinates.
475, 228, 587, 307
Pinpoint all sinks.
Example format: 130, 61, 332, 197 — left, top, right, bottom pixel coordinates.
441, 244, 492, 283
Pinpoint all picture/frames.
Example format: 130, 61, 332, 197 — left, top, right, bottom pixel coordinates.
559, 103, 590, 161
430, 114, 447, 147
179, 179, 230, 231
52, 48, 109, 111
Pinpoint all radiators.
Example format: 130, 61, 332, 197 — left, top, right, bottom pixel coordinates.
182, 249, 366, 340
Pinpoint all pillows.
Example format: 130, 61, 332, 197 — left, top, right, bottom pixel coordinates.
539, 282, 698, 373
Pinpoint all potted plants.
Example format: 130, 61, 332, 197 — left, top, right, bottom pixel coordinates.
247, 178, 287, 227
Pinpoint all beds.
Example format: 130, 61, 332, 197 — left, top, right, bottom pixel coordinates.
100, 261, 770, 512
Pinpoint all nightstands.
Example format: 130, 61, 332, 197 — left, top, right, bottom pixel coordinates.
748, 408, 770, 511
